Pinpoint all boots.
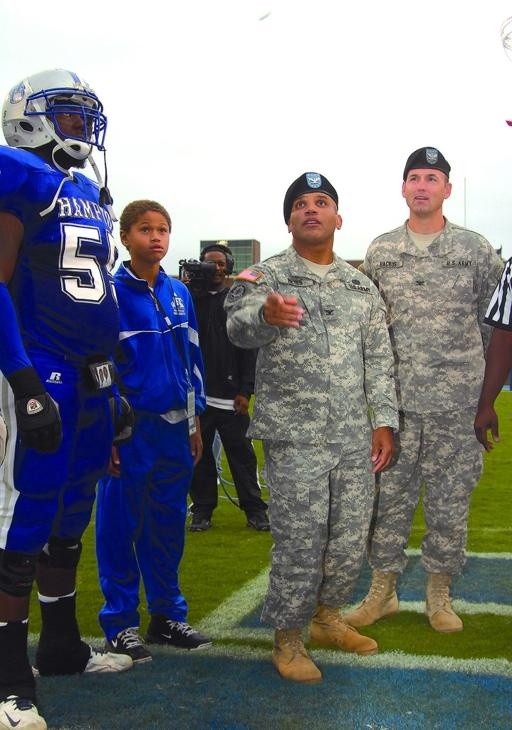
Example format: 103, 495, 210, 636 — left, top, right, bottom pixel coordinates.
271, 568, 463, 683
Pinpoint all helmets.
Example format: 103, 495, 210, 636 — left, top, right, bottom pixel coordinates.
3, 70, 106, 165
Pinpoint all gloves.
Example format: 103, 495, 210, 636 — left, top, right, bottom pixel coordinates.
110, 394, 134, 442
15, 392, 62, 453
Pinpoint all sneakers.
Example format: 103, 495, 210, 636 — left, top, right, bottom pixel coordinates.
247, 513, 271, 531
83, 615, 212, 673
0, 695, 47, 730
188, 509, 210, 531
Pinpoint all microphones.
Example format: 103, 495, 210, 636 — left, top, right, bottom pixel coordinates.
223, 268, 232, 274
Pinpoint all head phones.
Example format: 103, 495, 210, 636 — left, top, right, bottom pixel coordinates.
199, 244, 234, 269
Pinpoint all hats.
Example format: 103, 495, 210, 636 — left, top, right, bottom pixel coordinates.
284, 173, 338, 225
403, 147, 450, 181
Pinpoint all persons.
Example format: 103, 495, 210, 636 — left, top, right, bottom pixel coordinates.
474, 257, 512, 451
224, 171, 400, 684
97, 197, 213, 664
340, 147, 504, 634
0, 69, 136, 729
181, 245, 270, 532
